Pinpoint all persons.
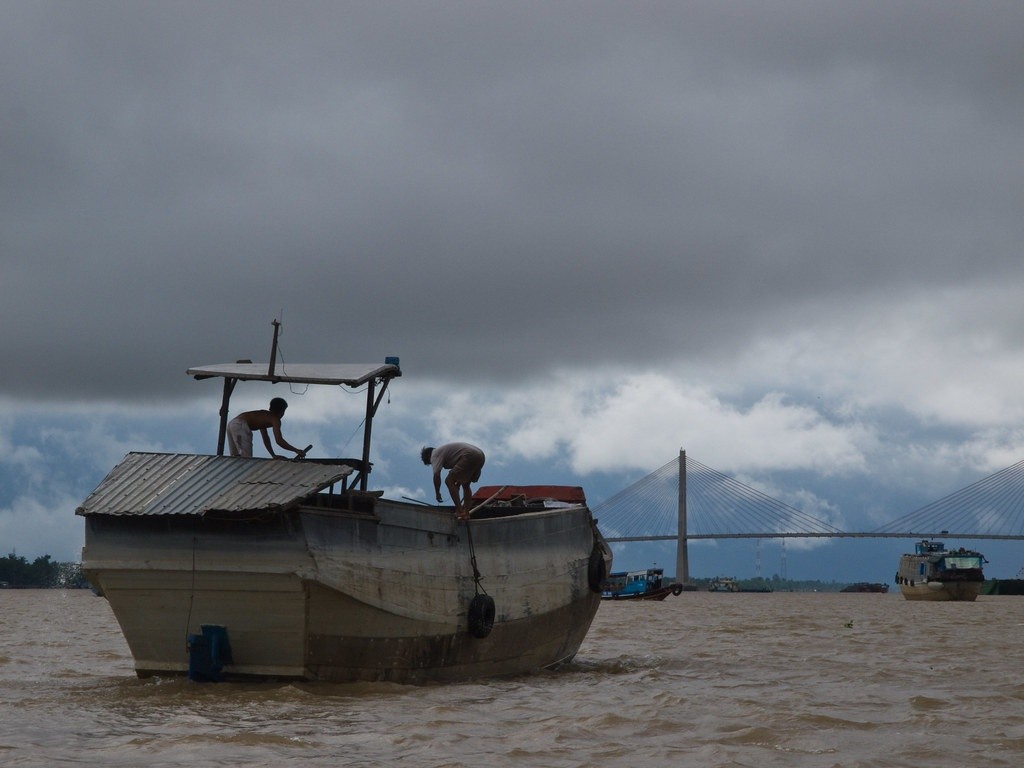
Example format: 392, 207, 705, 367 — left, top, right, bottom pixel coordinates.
422, 442, 486, 520
227, 397, 306, 459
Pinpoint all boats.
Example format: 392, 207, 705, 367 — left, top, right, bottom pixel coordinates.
600, 568, 682, 601
895, 540, 988, 602
709, 576, 735, 592
74, 318, 614, 683
